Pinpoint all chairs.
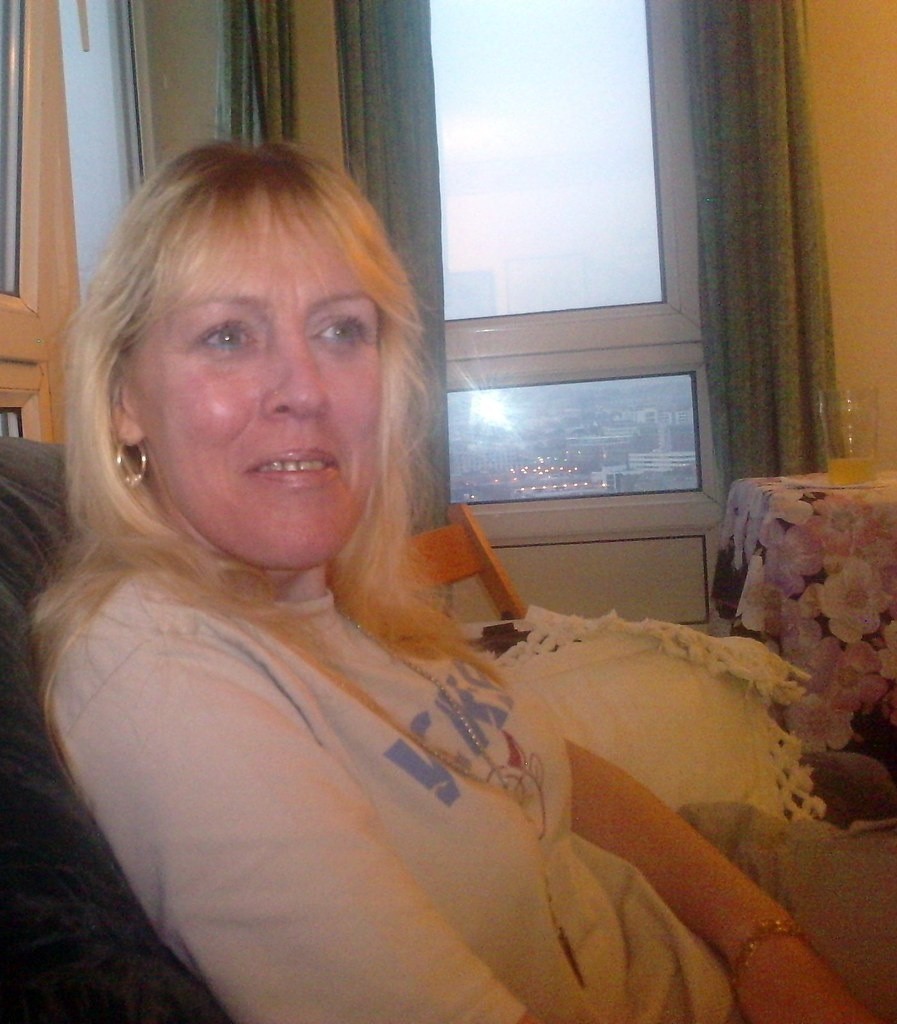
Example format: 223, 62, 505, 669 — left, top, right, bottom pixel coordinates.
371, 500, 528, 620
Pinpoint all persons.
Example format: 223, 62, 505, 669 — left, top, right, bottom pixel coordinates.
25, 139, 896, 1024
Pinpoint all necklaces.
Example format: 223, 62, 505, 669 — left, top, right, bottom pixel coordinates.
342, 611, 507, 789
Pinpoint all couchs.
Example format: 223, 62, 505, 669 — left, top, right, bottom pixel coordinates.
0, 438, 897, 1024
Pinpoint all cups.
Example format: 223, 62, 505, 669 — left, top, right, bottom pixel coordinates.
819, 386, 881, 486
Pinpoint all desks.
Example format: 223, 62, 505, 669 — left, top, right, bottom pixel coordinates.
709, 469, 897, 753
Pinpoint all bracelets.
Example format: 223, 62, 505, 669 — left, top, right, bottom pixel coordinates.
729, 919, 804, 998
